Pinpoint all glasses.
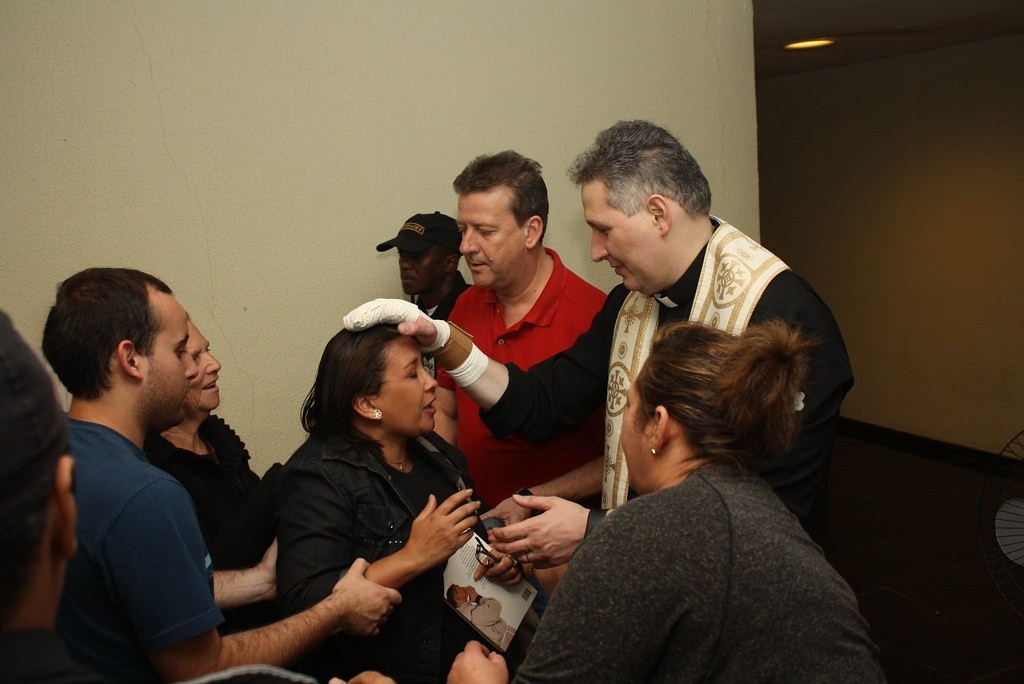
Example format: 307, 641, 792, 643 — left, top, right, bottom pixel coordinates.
474, 537, 500, 568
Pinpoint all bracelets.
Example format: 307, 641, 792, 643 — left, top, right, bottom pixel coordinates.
529, 563, 534, 578
515, 488, 542, 516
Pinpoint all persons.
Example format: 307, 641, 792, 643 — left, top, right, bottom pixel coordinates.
375, 212, 471, 321
0, 119, 887, 684
431, 151, 609, 530
447, 584, 515, 650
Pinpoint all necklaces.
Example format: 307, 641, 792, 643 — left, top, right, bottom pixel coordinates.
386, 453, 409, 471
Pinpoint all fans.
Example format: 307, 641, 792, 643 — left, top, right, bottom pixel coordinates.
977, 428, 1024, 620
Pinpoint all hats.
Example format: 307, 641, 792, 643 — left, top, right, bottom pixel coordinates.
375, 211, 464, 256
0, 310, 77, 497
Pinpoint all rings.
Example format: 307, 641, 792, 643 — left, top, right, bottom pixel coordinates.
526, 553, 530, 562
505, 554, 517, 566
